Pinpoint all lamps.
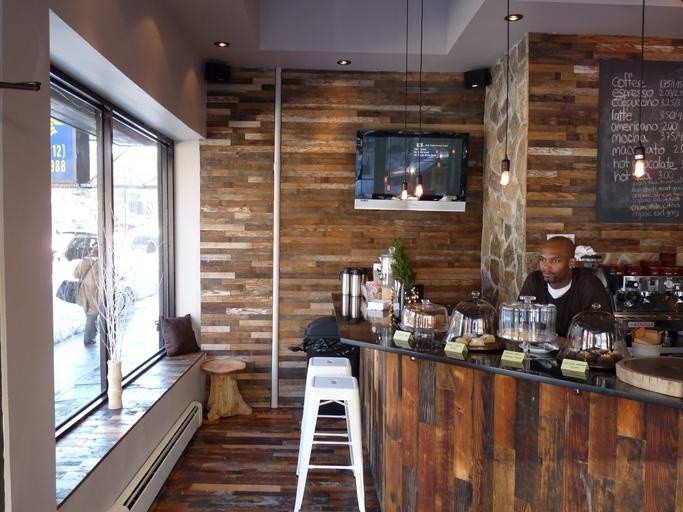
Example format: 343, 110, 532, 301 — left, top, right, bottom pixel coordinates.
401, 0, 408, 200
501, 0, 511, 185
416, 1, 423, 197
634, 0, 646, 179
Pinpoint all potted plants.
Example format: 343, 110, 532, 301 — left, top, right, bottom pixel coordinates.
90, 229, 135, 409
391, 234, 417, 323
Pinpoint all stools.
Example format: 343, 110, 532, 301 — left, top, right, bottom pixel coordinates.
202, 360, 252, 422
294, 375, 366, 512
296, 358, 358, 477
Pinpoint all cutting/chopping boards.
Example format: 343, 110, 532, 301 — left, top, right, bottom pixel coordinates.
616, 356, 683, 397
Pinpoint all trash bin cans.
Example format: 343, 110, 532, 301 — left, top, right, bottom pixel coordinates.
302, 315, 360, 418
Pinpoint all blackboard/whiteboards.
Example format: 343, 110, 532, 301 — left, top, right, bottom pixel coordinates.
597, 56, 683, 225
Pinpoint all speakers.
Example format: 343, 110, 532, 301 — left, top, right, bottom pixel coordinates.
463, 68, 493, 90
206, 62, 235, 83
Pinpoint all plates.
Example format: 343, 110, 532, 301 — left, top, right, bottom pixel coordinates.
556, 349, 617, 371
446, 332, 502, 352
518, 343, 559, 355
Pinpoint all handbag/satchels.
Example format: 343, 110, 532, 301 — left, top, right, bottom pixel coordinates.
56, 281, 81, 303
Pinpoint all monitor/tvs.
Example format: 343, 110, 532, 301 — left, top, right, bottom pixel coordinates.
355, 127, 470, 213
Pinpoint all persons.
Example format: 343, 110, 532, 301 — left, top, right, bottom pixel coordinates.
73, 247, 100, 346
516, 235, 613, 340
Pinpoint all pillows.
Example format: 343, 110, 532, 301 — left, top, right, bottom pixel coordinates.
160, 314, 201, 356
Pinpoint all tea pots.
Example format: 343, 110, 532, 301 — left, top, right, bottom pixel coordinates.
351, 297, 361, 321
349, 269, 361, 297
341, 296, 350, 320
338, 268, 349, 295
